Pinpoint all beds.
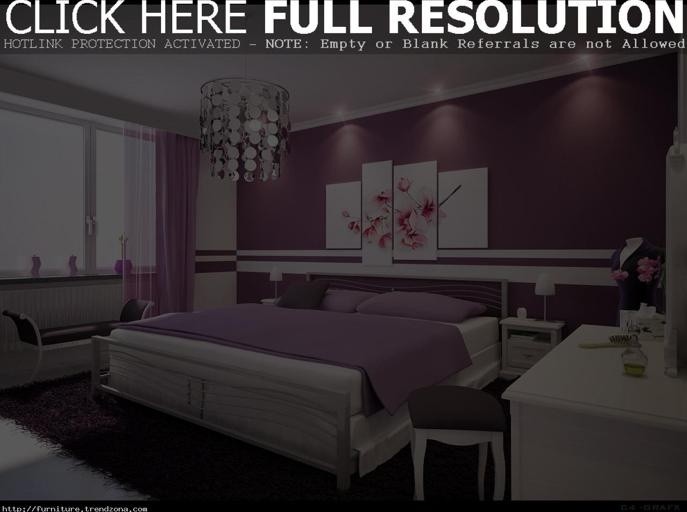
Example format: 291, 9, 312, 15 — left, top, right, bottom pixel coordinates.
90, 269, 507, 490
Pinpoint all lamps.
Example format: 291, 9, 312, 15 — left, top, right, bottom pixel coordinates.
266, 268, 285, 300
197, 55, 292, 186
534, 271, 555, 321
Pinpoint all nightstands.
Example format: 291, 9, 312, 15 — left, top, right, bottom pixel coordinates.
499, 318, 564, 380
259, 297, 279, 304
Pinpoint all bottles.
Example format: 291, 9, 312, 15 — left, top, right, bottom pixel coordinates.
619, 335, 649, 378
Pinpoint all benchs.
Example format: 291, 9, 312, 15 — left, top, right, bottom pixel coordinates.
2, 296, 157, 385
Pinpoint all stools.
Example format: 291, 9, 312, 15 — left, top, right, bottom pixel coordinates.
404, 385, 505, 501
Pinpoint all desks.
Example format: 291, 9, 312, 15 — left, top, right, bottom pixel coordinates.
501, 322, 686, 500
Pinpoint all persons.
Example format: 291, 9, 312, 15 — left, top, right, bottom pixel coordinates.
611, 236, 665, 310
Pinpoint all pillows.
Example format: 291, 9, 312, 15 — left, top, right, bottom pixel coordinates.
353, 291, 487, 324
320, 288, 378, 313
274, 282, 328, 308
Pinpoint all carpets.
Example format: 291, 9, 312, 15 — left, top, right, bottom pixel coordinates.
0, 364, 518, 502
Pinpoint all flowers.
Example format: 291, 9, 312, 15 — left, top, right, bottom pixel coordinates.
638, 257, 659, 283
608, 269, 628, 282
338, 174, 463, 256
